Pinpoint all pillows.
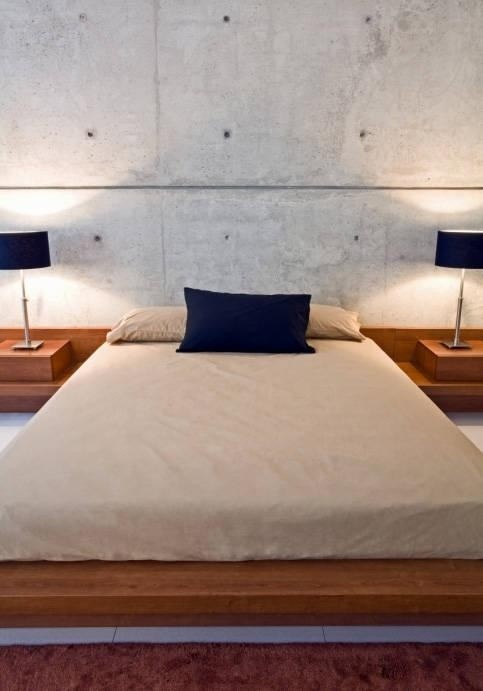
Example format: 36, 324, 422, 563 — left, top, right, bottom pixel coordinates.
107, 308, 189, 344
176, 285, 313, 355
294, 306, 364, 341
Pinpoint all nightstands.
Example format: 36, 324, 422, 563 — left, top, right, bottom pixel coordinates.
394, 340, 483, 408
0, 340, 78, 413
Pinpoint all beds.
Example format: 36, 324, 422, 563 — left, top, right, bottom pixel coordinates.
0, 305, 482, 627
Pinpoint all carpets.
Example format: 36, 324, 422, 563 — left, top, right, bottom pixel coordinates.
0, 641, 483, 690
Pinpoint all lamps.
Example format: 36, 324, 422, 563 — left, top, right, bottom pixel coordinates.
0, 231, 52, 353
436, 230, 483, 351
